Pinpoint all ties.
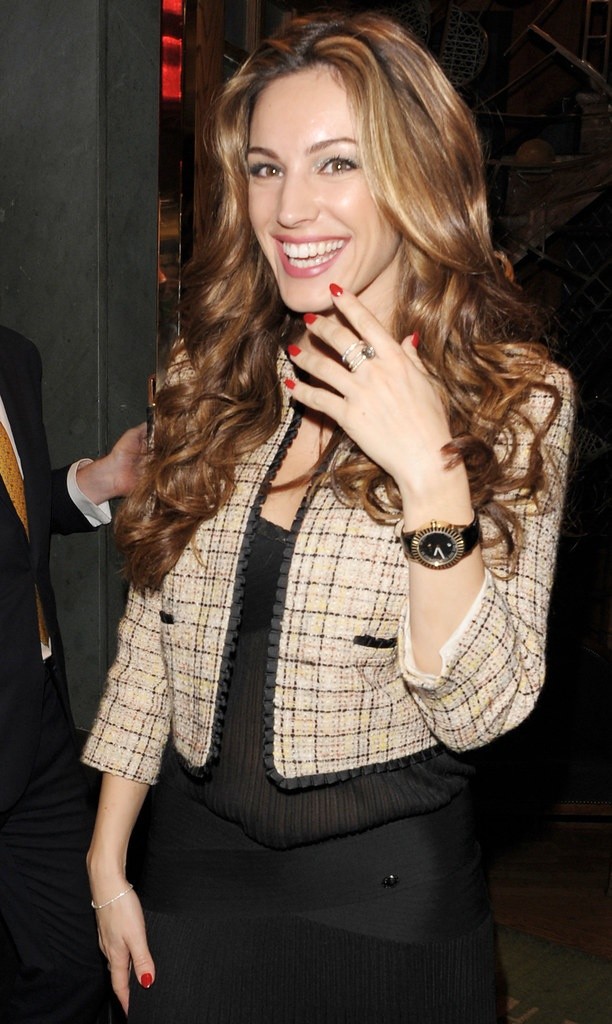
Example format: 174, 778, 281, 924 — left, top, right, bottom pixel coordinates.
0, 421, 50, 648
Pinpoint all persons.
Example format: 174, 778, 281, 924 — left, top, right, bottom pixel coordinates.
78, 14, 574, 1024
0, 323, 155, 1024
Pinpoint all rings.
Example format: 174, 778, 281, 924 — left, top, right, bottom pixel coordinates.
342, 341, 375, 372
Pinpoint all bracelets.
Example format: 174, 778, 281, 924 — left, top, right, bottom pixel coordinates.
89, 884, 134, 909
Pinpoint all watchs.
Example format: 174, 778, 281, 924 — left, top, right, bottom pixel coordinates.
401, 511, 480, 569
